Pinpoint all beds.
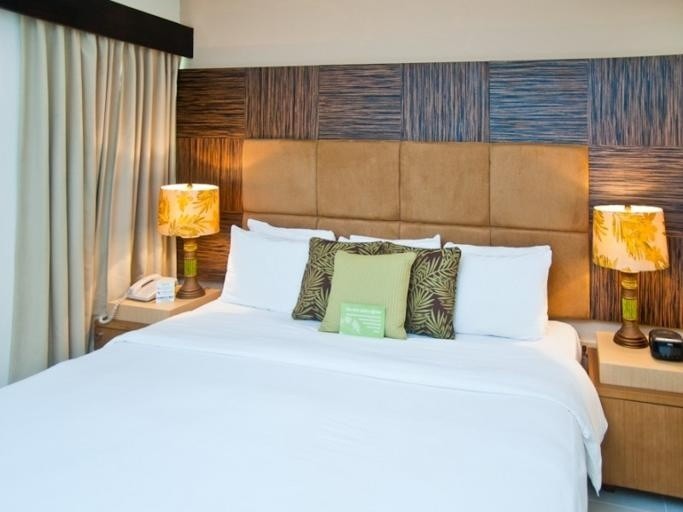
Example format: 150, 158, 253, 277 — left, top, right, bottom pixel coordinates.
0, 137, 594, 512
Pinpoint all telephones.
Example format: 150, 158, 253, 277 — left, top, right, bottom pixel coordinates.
126, 274, 176, 302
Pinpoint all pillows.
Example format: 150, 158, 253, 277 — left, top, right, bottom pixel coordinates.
348, 235, 440, 248
222, 225, 311, 314
246, 217, 334, 239
441, 242, 553, 343
294, 237, 381, 321
320, 249, 417, 341
378, 241, 460, 341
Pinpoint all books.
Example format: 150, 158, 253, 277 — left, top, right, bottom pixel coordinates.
338, 302, 385, 338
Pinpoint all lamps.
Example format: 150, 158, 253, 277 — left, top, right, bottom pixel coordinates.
155, 183, 221, 301
590, 204, 672, 349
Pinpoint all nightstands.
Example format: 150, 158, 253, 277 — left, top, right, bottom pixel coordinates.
92, 286, 222, 350
588, 331, 683, 500
